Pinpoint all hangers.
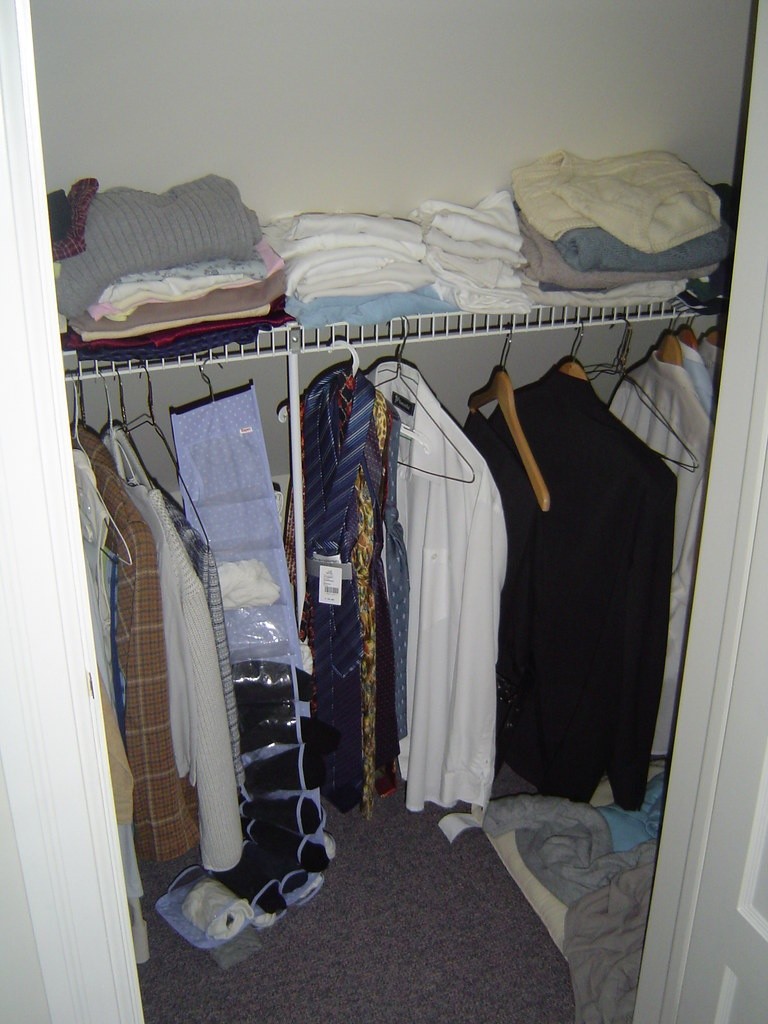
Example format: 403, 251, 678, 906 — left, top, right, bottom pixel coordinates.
66, 313, 475, 565
468, 322, 554, 512
559, 316, 724, 473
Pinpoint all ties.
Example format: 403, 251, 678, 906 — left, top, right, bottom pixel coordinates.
283, 366, 410, 815
